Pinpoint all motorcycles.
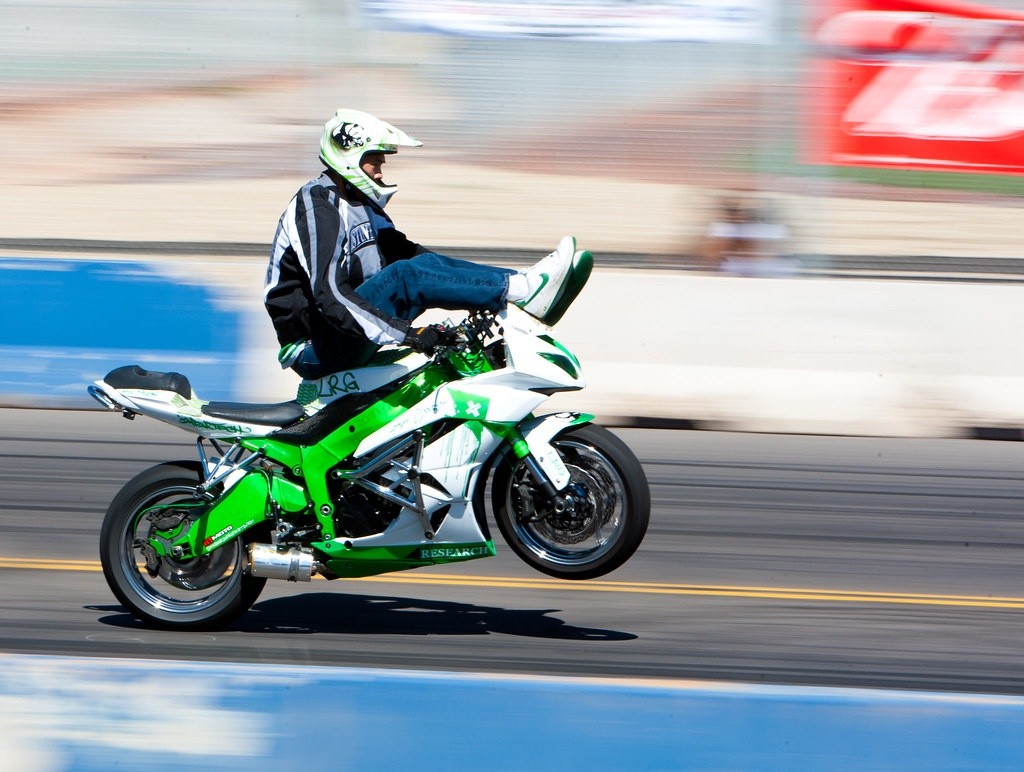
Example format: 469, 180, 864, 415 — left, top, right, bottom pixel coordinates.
88, 301, 651, 631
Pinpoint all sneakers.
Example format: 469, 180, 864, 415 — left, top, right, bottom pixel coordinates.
513, 236, 576, 318
544, 250, 593, 327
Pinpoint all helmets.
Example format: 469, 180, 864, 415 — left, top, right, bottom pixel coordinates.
319, 109, 422, 210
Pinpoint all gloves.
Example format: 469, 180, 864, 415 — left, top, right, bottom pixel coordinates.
404, 326, 441, 356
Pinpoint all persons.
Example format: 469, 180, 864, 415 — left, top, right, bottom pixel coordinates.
264, 107, 595, 380
